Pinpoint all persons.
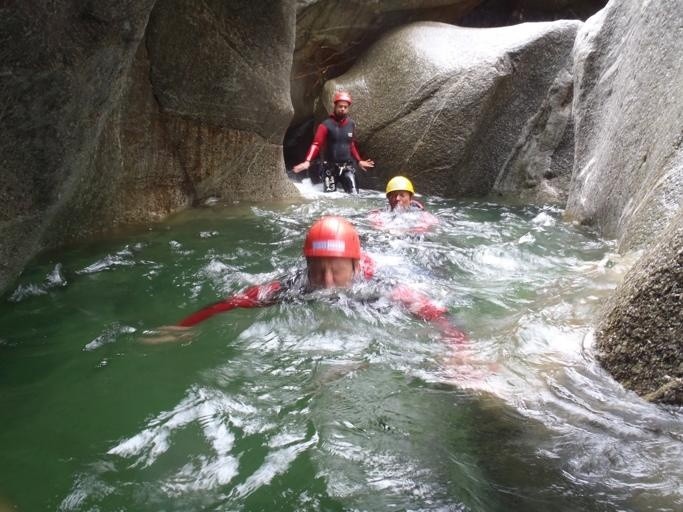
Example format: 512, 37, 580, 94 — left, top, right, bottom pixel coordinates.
288, 90, 376, 197
135, 214, 502, 391
359, 174, 440, 236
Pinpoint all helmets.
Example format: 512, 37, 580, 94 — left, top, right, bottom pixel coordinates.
303, 217, 361, 260
334, 91, 352, 105
385, 176, 414, 197
